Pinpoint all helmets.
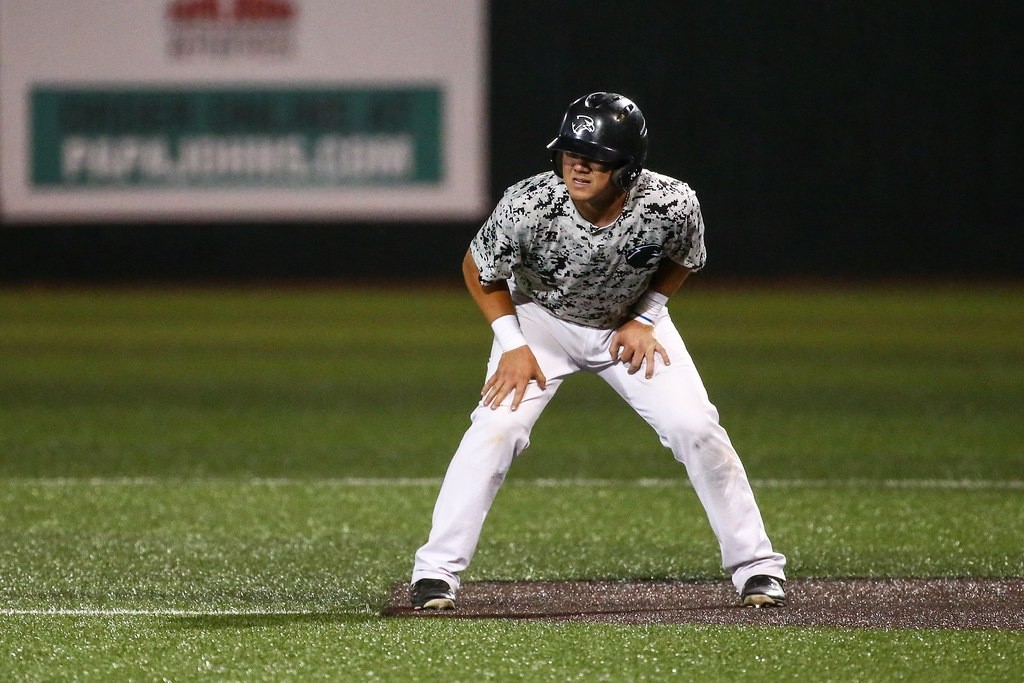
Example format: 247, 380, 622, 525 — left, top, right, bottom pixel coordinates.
546, 92, 650, 193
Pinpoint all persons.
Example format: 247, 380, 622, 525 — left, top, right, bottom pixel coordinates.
407, 92, 787, 610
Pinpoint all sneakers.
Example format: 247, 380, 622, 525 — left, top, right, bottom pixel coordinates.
741, 575, 786, 608
410, 578, 456, 611
